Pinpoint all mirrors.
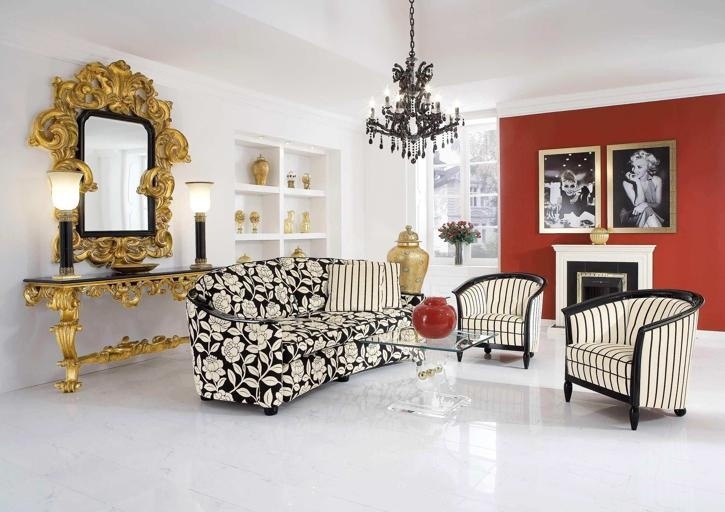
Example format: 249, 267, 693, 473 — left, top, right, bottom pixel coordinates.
24, 59, 190, 269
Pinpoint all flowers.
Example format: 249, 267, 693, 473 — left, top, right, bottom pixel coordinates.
438, 220, 481, 246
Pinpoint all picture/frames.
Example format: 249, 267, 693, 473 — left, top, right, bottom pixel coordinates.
538, 145, 602, 235
606, 138, 677, 235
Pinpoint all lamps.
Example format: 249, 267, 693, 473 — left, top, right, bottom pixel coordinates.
46, 171, 85, 279
365, 0, 465, 164
185, 182, 215, 268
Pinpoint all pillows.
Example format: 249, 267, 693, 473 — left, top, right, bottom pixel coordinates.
348, 259, 402, 310
325, 262, 383, 312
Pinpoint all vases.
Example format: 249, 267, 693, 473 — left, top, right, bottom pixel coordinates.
454, 241, 463, 265
412, 296, 457, 339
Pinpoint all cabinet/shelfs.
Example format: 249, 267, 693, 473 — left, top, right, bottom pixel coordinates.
214, 129, 332, 268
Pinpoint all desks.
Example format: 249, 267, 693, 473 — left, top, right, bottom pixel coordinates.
551, 244, 657, 329
22, 263, 221, 392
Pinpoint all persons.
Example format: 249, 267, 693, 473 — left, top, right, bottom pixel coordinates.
554, 170, 595, 229
619, 149, 664, 227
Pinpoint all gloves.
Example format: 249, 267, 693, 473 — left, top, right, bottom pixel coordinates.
581, 186, 590, 212
559, 187, 567, 220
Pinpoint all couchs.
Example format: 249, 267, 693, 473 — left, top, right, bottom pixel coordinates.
561, 288, 706, 431
451, 271, 548, 370
185, 256, 425, 416
